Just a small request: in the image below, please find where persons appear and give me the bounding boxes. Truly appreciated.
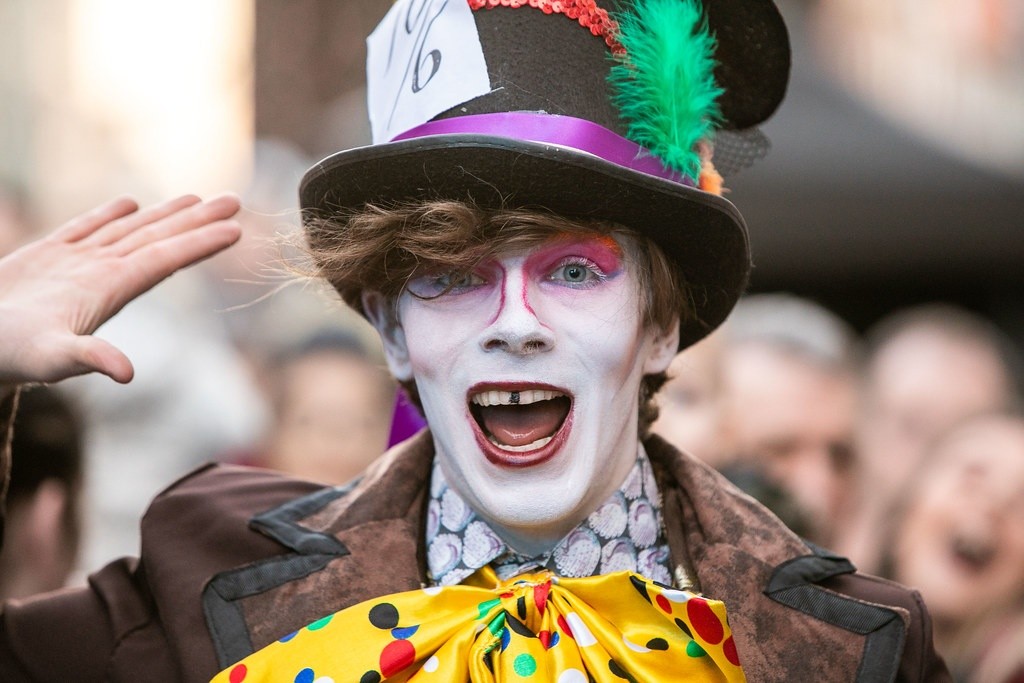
[0,0,955,683]
[0,288,1024,683]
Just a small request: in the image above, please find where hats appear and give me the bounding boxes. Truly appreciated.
[298,0,792,354]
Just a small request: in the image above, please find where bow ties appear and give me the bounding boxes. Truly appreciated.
[208,565,747,683]
